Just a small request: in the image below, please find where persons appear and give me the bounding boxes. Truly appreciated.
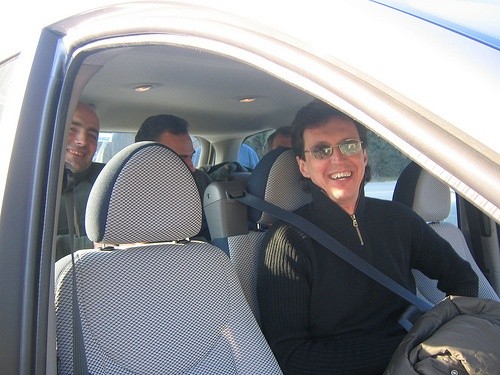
[54,100,107,262]
[264,126,295,155]
[135,114,214,244]
[255,98,480,375]
[192,142,259,173]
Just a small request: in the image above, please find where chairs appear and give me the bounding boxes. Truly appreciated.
[391,160,500,306]
[55,140,285,375]
[204,146,317,330]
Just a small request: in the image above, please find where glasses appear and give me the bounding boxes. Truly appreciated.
[303,139,364,161]
